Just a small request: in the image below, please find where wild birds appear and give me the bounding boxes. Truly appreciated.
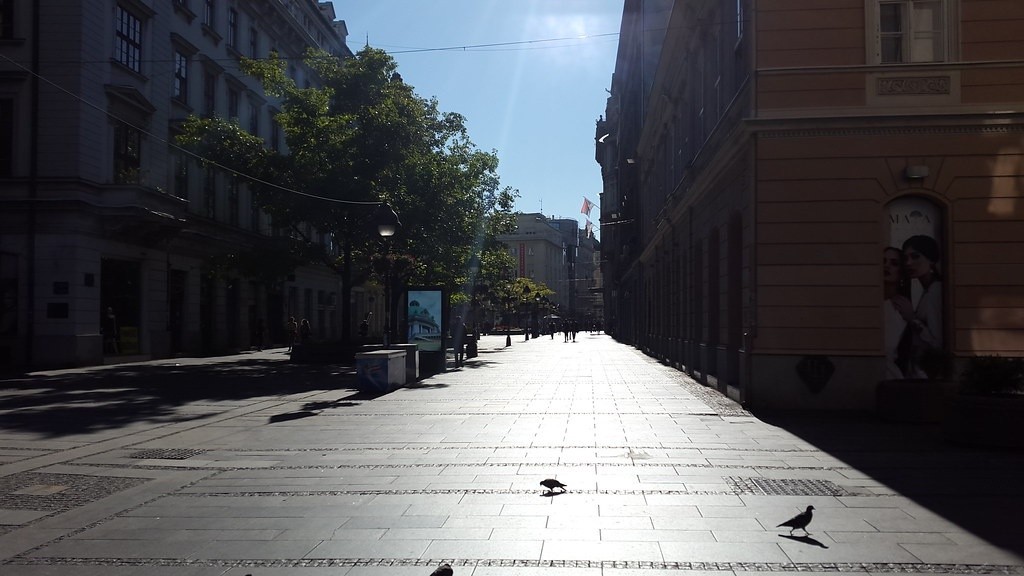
[430,563,453,576]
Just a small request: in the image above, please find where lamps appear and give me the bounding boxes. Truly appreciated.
[905,165,930,179]
[660,90,671,102]
[626,150,637,164]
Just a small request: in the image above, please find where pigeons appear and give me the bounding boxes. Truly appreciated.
[776,505,816,535]
[540,479,567,493]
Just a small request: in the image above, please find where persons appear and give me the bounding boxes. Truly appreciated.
[883,234,944,378]
[584,319,602,333]
[359,319,368,338]
[287,317,311,351]
[549,321,563,340]
[449,315,467,368]
[563,318,578,343]
[101,307,119,355]
[254,318,265,352]
